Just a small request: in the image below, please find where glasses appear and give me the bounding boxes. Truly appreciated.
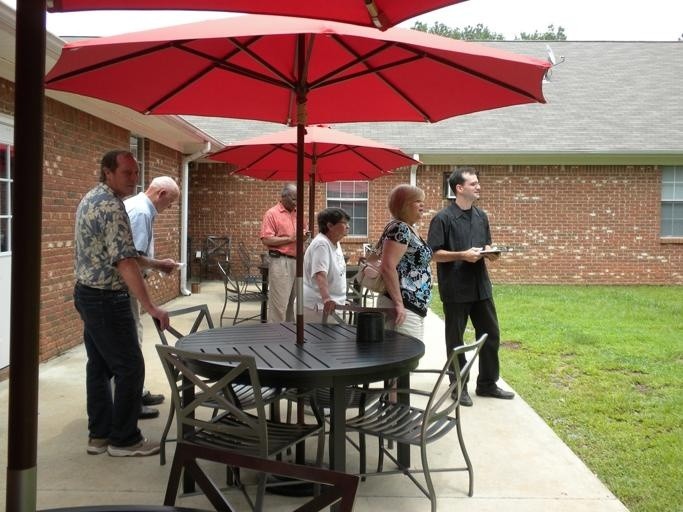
[338,221,347,226]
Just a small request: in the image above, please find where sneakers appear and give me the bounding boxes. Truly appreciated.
[107,436,160,457]
[87,436,107,455]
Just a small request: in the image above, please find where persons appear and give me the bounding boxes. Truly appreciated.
[259,183,311,324]
[124,176,181,418]
[71,150,170,458]
[427,166,516,407]
[375,183,435,408]
[303,206,352,418]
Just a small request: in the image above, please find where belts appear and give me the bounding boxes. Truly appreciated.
[280,253,296,259]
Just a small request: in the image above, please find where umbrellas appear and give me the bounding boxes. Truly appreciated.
[202,123,422,237]
[43,14,552,345]
[47,0,473,34]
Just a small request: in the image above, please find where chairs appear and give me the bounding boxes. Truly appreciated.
[152,304,289,486]
[347,332,487,512]
[217,261,269,327]
[286,300,395,463]
[155,344,326,512]
[162,441,361,512]
[235,241,263,294]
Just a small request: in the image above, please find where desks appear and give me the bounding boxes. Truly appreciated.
[174,322,425,474]
[258,263,363,325]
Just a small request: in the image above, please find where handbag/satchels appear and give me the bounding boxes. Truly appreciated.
[355,220,401,294]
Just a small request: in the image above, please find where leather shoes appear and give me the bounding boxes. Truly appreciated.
[139,407,159,418]
[476,384,514,399]
[141,392,164,405]
[451,385,473,407]
